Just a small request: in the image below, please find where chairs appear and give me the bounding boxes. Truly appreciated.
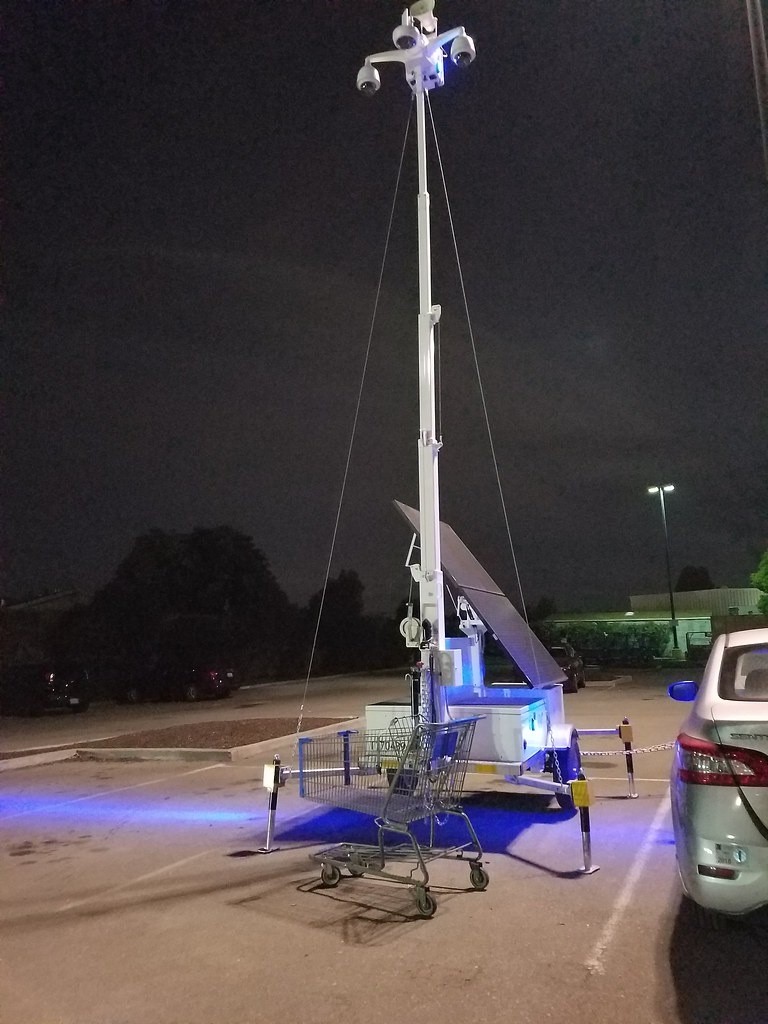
[745,669,768,699]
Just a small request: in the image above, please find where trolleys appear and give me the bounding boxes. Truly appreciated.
[297,712,490,919]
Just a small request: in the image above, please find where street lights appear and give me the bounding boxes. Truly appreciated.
[646,479,685,658]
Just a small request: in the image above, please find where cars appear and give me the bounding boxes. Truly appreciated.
[665,626,767,919]
[0,664,90,720]
[513,642,585,693]
[116,665,233,701]
[576,648,610,668]
